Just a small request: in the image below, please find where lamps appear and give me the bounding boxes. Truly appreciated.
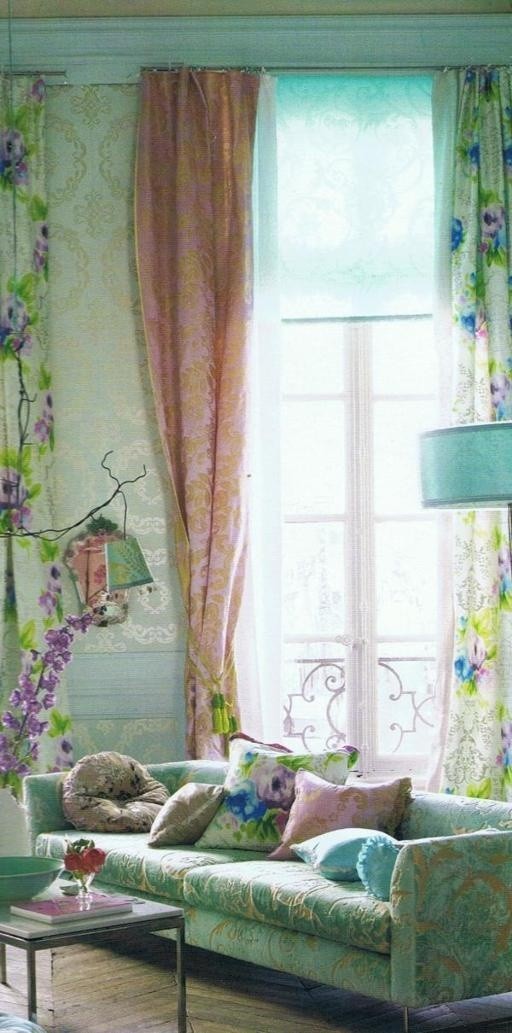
[410,421,512,558]
[103,489,154,594]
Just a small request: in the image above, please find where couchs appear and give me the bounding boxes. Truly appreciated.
[21,756,512,1033]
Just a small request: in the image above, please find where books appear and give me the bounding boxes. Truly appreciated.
[9,890,134,926]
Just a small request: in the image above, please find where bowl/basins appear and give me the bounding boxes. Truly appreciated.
[0,856,68,902]
